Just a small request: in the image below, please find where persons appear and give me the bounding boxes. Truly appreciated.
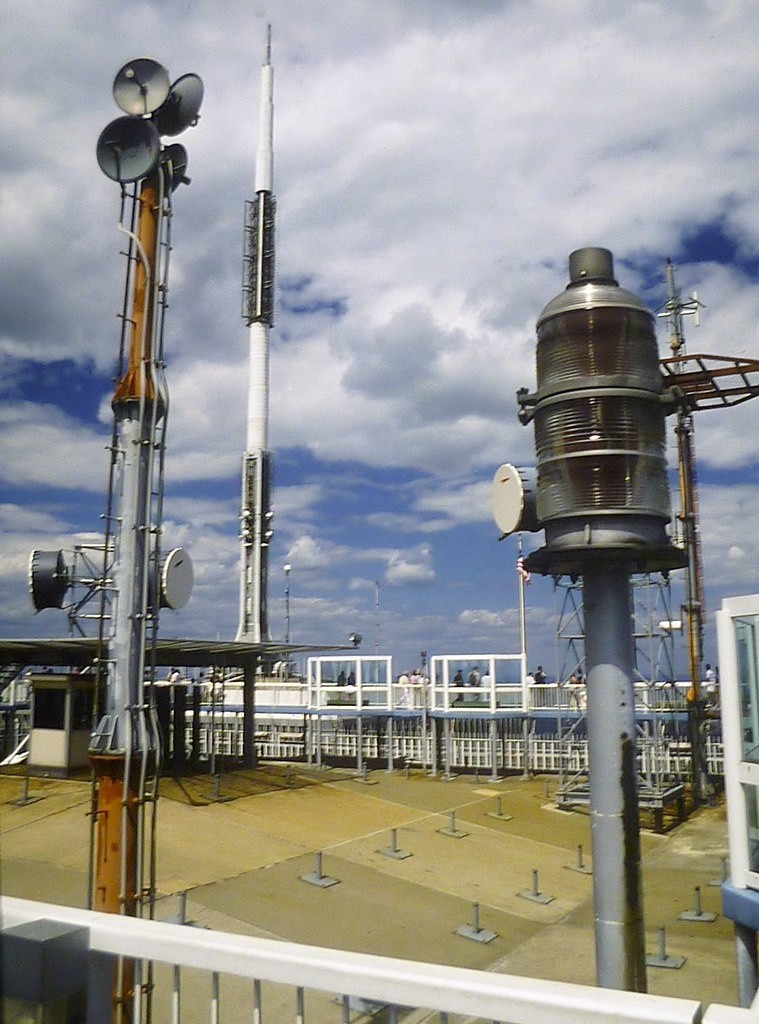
[702,664,717,704]
[26,659,587,707]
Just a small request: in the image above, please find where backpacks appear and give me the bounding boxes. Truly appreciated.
[535,671,543,683]
[468,672,475,686]
